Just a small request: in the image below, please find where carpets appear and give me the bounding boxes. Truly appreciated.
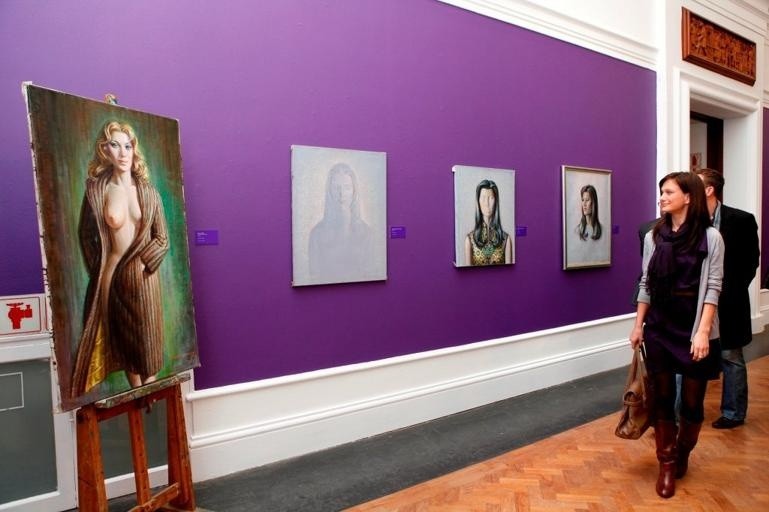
[61,321,769,512]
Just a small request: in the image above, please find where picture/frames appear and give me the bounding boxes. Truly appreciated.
[561,163,613,270]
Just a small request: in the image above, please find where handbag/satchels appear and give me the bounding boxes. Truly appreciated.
[615,342,651,439]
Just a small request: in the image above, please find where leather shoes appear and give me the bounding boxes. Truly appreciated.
[712,416,744,428]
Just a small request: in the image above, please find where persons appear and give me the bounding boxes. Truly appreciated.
[464,179,513,265]
[572,185,607,260]
[632,202,683,438]
[694,168,760,430]
[69,119,170,399]
[629,171,725,498]
[309,160,373,282]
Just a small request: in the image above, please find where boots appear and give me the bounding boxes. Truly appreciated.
[654,417,703,498]
